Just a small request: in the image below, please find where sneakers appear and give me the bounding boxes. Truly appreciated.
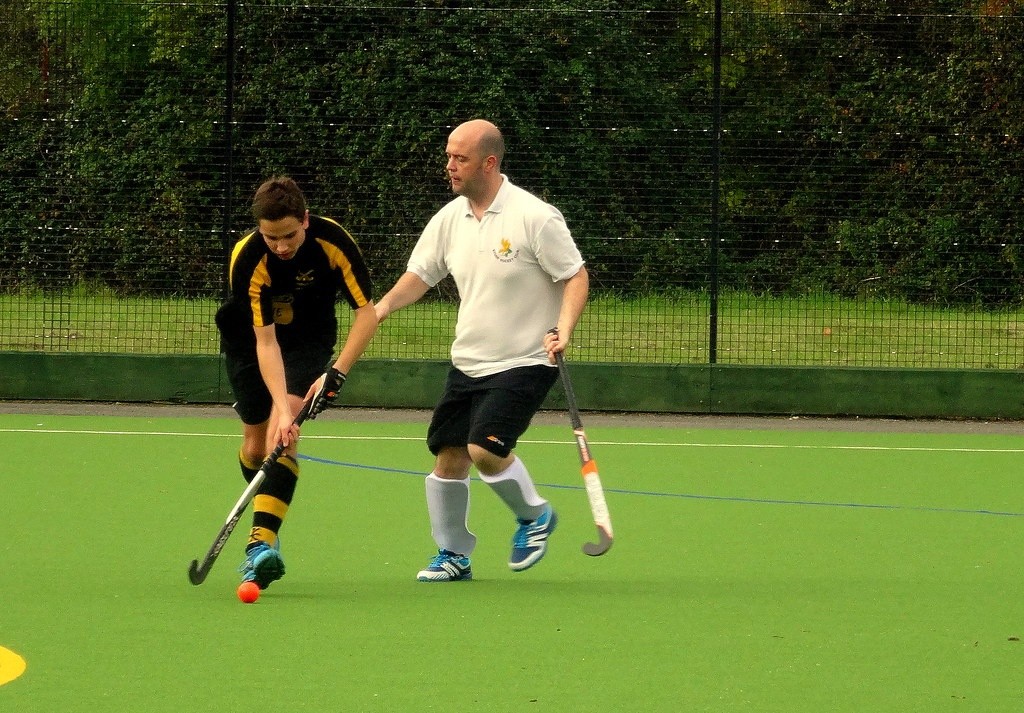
[238,541,286,589]
[506,502,557,571]
[416,549,472,581]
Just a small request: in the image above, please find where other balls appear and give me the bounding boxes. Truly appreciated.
[237,582,262,602]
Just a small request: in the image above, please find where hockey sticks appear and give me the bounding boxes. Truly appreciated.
[549,327,614,556]
[188,399,312,587]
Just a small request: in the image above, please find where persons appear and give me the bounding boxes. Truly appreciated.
[213,177,378,590]
[373,119,589,579]
